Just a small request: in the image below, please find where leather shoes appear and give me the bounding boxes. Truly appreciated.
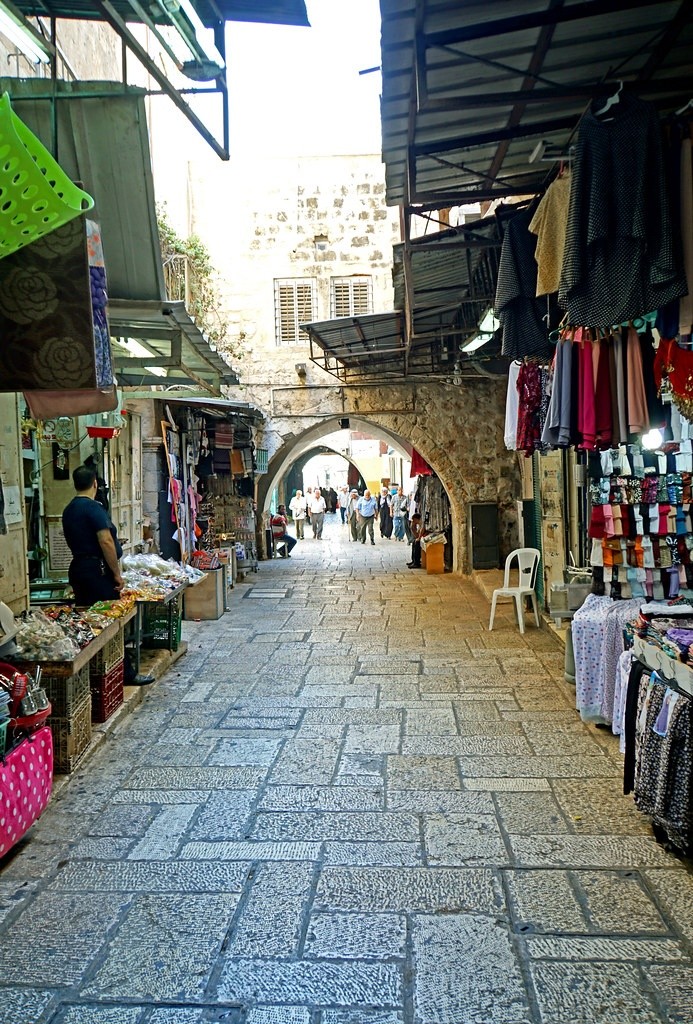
[125,673,155,686]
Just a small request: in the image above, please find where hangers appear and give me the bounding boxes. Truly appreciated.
[524,355,552,370]
[675,98,693,115]
[558,319,633,342]
[593,78,624,122]
[563,146,576,174]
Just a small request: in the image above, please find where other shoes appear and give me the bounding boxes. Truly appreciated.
[297,536,299,539]
[399,538,404,542]
[282,550,291,557]
[381,534,383,538]
[406,561,414,565]
[371,541,375,545]
[277,549,284,556]
[408,563,420,568]
[361,540,365,544]
[317,536,322,540]
[313,534,316,538]
[300,536,304,539]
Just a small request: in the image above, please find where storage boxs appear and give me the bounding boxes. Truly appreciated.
[38,662,90,717]
[88,625,124,677]
[143,592,182,652]
[46,695,93,775]
[184,568,224,620]
[91,661,123,723]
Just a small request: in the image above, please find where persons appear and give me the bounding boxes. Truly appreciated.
[271,504,297,557]
[289,486,338,540]
[62,465,125,607]
[338,484,432,569]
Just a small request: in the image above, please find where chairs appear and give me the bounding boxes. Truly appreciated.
[489,548,540,634]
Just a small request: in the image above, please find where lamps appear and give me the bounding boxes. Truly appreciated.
[338,417,350,429]
[295,363,307,377]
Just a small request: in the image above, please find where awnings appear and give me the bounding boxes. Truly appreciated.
[299,309,442,368]
[106,297,241,387]
[1,76,167,300]
[379,1,693,206]
[392,206,530,308]
[1,0,310,28]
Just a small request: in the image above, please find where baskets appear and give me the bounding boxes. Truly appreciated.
[0,89,95,258]
